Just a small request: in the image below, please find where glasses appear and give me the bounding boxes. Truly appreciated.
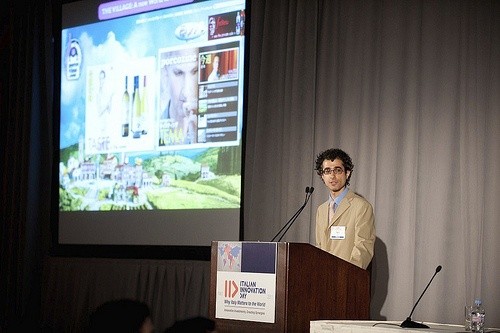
[322,167,347,175]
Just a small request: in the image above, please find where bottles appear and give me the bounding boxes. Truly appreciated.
[471,299,486,333]
[121,74,150,139]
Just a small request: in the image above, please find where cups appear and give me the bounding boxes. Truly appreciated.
[465,305,473,331]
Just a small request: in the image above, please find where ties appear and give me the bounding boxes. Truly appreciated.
[329,201,335,225]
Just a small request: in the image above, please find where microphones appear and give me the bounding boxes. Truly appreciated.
[400,265,442,328]
[270,187,314,242]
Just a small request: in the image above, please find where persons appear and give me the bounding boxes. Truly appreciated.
[209,16,217,36]
[82,297,155,333]
[207,54,222,82]
[162,315,216,333]
[159,47,199,142]
[315,148,375,270]
